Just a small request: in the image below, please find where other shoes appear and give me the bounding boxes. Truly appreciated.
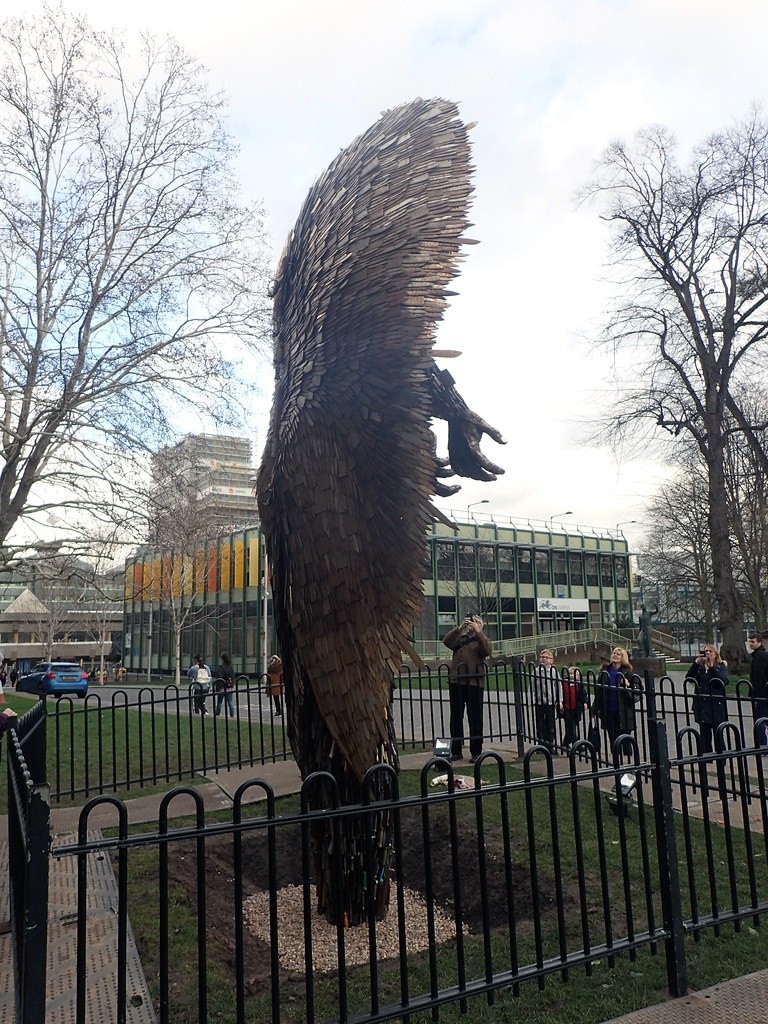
[449,753,463,761]
[274,712,282,717]
[469,755,478,763]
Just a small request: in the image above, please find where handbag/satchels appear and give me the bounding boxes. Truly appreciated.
[588,722,601,752]
[622,672,640,704]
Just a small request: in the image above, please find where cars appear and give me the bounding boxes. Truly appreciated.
[15,663,88,699]
[103,665,126,675]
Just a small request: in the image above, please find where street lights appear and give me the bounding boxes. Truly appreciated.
[468,500,489,519]
[551,512,573,528]
[617,521,636,535]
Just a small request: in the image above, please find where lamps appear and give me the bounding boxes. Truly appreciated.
[431,736,455,770]
[604,773,636,819]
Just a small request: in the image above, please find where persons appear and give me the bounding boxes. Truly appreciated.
[0,670,8,687]
[10,668,18,687]
[87,665,127,683]
[588,646,645,757]
[265,655,284,717]
[685,644,730,765]
[442,613,493,764]
[556,666,590,752]
[747,633,768,756]
[187,653,212,715]
[526,649,563,756]
[215,653,235,717]
[637,602,659,657]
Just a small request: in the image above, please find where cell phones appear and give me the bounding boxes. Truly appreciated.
[467,613,474,623]
[700,651,706,657]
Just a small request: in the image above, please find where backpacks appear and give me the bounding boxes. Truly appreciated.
[195,665,211,684]
[219,665,235,688]
